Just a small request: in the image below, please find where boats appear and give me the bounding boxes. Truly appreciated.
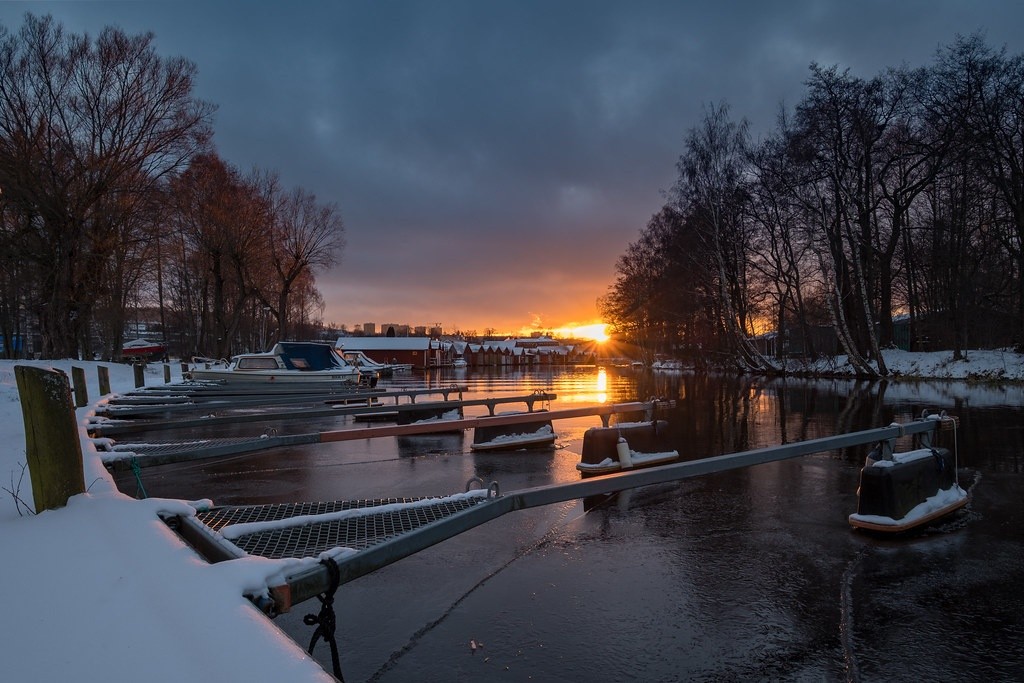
[186,341,413,384]
[652,361,695,369]
[575,420,680,476]
[110,339,166,361]
[470,408,559,453]
[610,360,644,368]
[324,398,384,409]
[847,448,984,532]
[452,359,467,367]
